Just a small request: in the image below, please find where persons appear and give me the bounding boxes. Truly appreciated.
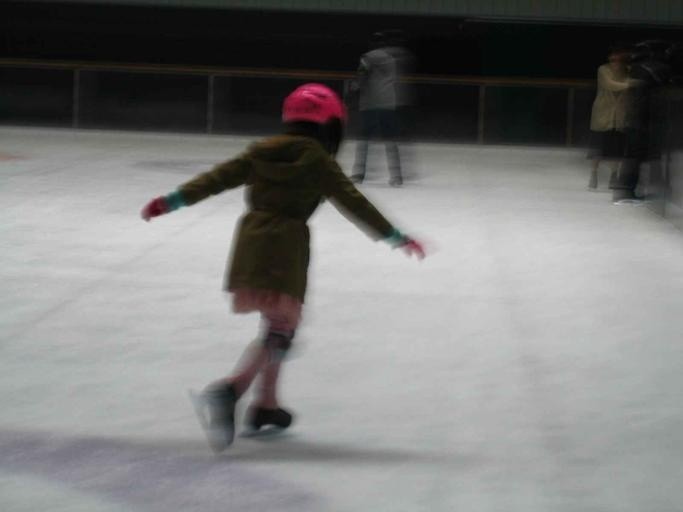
[611,38,665,203]
[585,43,644,190]
[139,81,428,450]
[345,31,403,187]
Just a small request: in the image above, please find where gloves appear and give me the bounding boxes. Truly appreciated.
[141,190,186,222]
[383,227,425,262]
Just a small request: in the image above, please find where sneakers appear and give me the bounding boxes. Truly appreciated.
[243,405,292,427]
[389,178,403,186]
[204,379,235,443]
[348,174,364,181]
[588,175,644,201]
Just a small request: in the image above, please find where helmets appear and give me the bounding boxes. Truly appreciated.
[281,83,351,131]
